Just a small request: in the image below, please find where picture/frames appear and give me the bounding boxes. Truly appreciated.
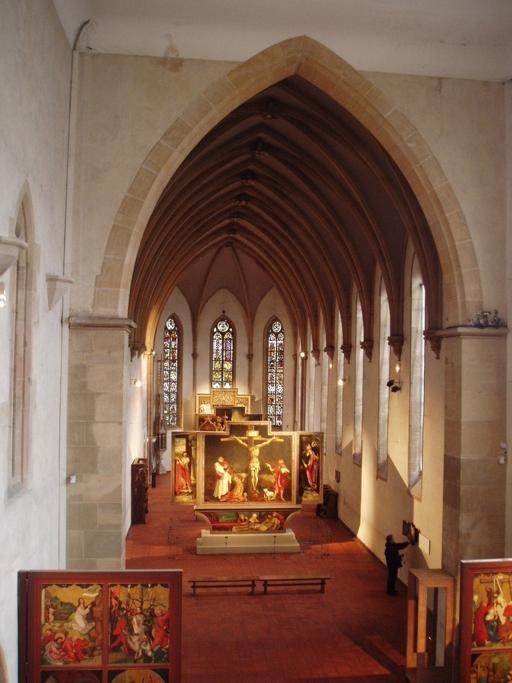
[195,422,301,512]
[17,571,182,683]
[297,431,323,505]
[460,557,512,683]
[168,429,201,507]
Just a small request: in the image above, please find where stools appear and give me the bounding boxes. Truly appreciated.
[406,566,455,683]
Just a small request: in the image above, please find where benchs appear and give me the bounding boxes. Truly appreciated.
[188,576,256,596]
[257,575,330,594]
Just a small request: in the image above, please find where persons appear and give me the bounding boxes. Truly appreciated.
[45,589,169,664]
[231,434,280,490]
[265,458,290,502]
[213,456,232,499]
[472,592,512,647]
[175,451,192,493]
[208,420,225,431]
[384,534,411,596]
[299,441,312,491]
[219,460,248,502]
[302,446,319,490]
